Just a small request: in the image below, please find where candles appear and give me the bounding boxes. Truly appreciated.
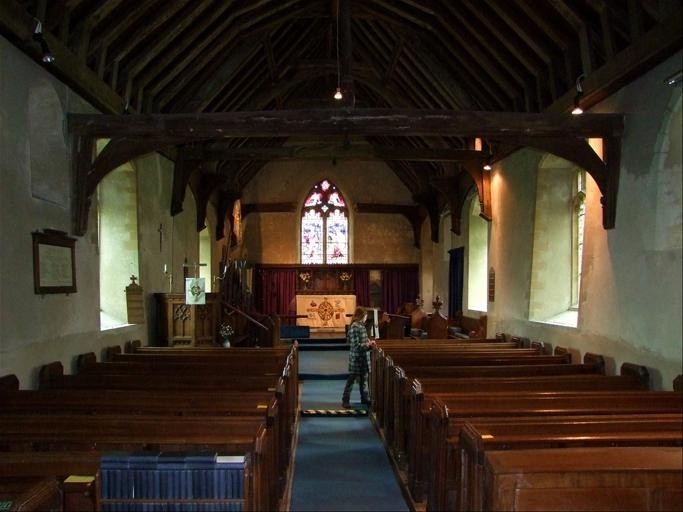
[223,265,228,275]
[164,264,168,274]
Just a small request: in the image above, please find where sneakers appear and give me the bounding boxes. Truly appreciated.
[340,402,350,408]
[361,400,369,404]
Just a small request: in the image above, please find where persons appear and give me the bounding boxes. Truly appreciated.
[342,305,376,410]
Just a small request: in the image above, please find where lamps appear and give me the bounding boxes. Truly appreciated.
[332,0,343,100]
[571,73,584,115]
[483,154,493,172]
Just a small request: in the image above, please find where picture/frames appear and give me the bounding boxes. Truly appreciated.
[30,227,78,296]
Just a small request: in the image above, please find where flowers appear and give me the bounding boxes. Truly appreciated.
[218,320,235,337]
